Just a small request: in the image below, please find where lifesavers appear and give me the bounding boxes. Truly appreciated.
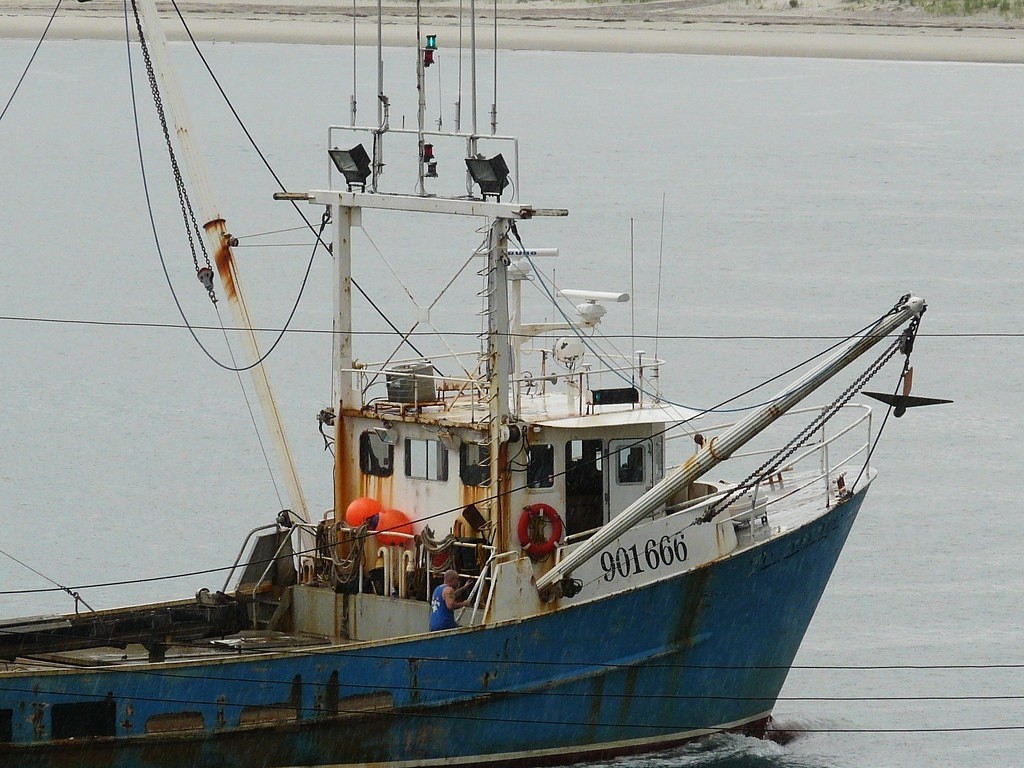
[518,503,562,555]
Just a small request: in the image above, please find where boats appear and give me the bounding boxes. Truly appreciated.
[0,1,955,767]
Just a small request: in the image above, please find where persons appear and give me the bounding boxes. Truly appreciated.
[429,569,473,633]
